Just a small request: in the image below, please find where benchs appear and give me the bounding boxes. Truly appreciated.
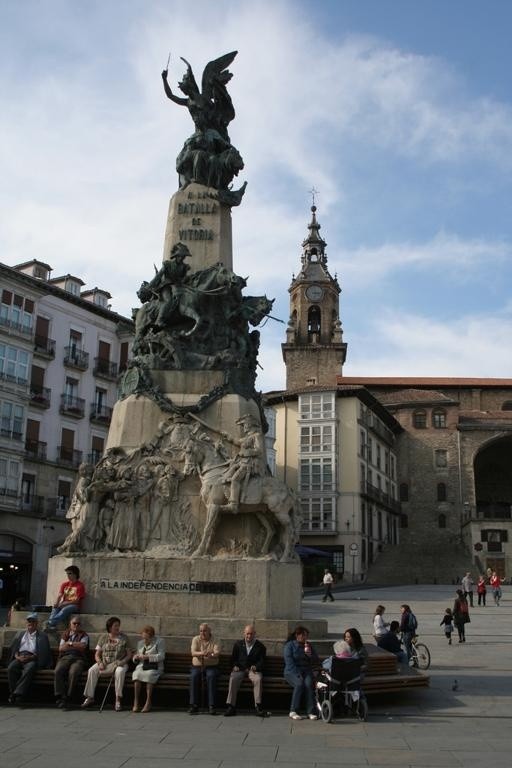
[0,655,430,692]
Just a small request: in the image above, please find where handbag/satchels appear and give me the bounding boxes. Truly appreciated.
[142,661,158,670]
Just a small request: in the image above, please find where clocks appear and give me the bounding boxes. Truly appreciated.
[306,285,323,301]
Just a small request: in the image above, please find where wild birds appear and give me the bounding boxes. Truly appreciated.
[452,678,458,691]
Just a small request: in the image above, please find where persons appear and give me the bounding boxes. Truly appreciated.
[400,604,418,659]
[131,626,166,713]
[441,567,506,645]
[81,617,131,711]
[373,605,387,643]
[54,617,89,704]
[319,569,334,602]
[224,624,264,716]
[161,70,214,134]
[283,624,368,720]
[220,415,263,514]
[57,447,180,553]
[144,241,192,328]
[188,623,220,715]
[7,614,51,703]
[44,565,85,632]
[378,621,408,665]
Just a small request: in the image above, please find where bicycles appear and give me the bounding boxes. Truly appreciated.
[398,630,431,670]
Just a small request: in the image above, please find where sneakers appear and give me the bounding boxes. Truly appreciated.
[115,700,120,711]
[187,706,198,713]
[290,712,301,719]
[208,707,215,714]
[255,710,266,715]
[134,702,140,711]
[83,696,93,707]
[142,701,151,712]
[225,709,235,716]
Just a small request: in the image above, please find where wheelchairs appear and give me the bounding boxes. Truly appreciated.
[313,656,370,723]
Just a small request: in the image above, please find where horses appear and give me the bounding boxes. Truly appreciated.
[184,433,304,562]
[131,262,276,370]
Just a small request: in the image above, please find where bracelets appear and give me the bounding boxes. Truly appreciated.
[69,641,72,646]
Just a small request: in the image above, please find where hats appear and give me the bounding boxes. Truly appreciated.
[27,613,36,619]
[100,663,116,676]
